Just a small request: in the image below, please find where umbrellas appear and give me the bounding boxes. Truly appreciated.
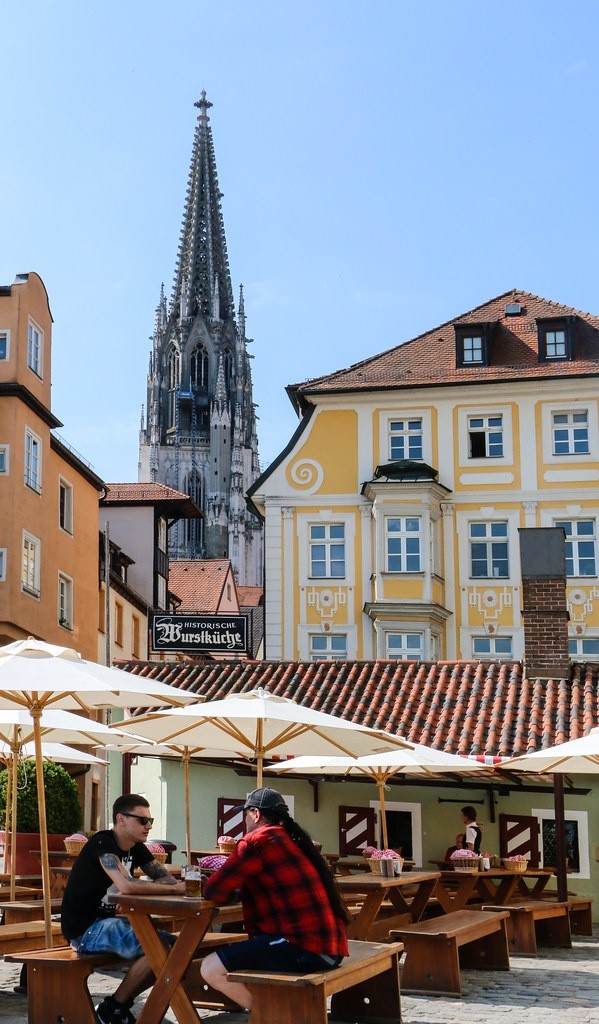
[0,635,599,952]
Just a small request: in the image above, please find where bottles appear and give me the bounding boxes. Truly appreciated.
[494,854,498,867]
[479,853,484,872]
[386,855,394,877]
[379,854,387,876]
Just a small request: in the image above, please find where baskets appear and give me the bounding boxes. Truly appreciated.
[131,853,168,871]
[448,856,482,873]
[199,868,218,896]
[63,839,88,854]
[502,858,529,873]
[218,842,237,853]
[366,857,404,875]
[315,844,323,853]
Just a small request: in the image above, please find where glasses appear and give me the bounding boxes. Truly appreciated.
[113,811,154,826]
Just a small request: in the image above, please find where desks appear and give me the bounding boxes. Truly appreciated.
[433,868,554,912]
[26,850,77,867]
[182,849,224,868]
[106,891,244,1024]
[50,866,73,899]
[331,871,442,941]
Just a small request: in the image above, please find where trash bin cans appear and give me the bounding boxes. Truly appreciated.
[147,840,177,864]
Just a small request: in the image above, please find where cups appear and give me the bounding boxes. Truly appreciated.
[180,864,195,882]
[184,866,202,898]
[393,861,401,877]
[483,858,489,870]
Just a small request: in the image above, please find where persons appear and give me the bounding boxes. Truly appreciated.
[388,843,413,872]
[445,834,465,861]
[60,794,208,1024]
[460,806,482,856]
[200,787,351,1014]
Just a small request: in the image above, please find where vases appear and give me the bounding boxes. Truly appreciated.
[63,840,87,854]
[365,858,404,876]
[153,852,168,866]
[363,852,372,858]
[218,841,236,852]
[503,861,529,872]
[449,857,481,874]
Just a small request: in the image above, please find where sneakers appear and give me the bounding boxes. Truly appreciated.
[93,1000,132,1024]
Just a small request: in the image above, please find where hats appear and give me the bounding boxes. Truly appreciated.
[232,786,288,813]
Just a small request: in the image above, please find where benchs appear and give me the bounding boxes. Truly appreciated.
[0,860,594,1024]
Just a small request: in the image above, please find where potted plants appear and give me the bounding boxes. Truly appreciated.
[0,759,83,875]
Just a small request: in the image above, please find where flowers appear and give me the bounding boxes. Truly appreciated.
[197,855,228,869]
[65,834,88,841]
[372,850,401,860]
[217,836,235,843]
[506,854,527,862]
[451,849,477,857]
[144,843,165,853]
[362,846,378,853]
